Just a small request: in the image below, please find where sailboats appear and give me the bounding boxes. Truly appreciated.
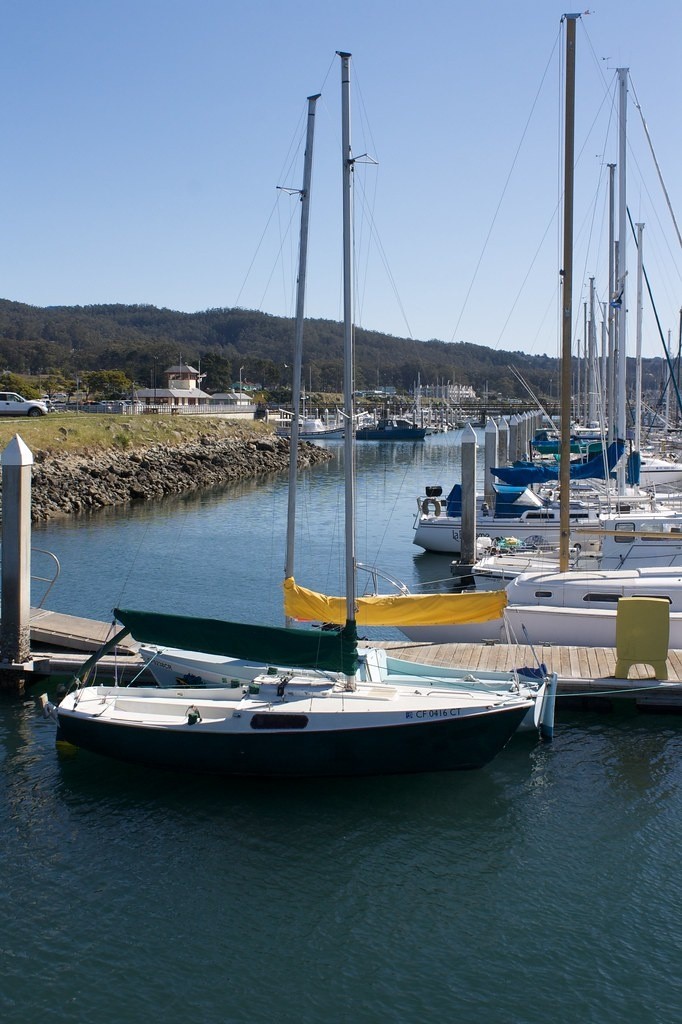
[53,46,535,779]
[390,9,682,650]
[108,90,562,744]
[272,370,503,441]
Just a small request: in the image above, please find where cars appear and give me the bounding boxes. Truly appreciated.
[0,392,48,417]
[37,398,143,412]
[53,392,67,398]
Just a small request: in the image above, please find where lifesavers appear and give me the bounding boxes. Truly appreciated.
[421,497,442,518]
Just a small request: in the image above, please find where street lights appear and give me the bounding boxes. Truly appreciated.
[153,355,159,397]
[240,365,245,405]
